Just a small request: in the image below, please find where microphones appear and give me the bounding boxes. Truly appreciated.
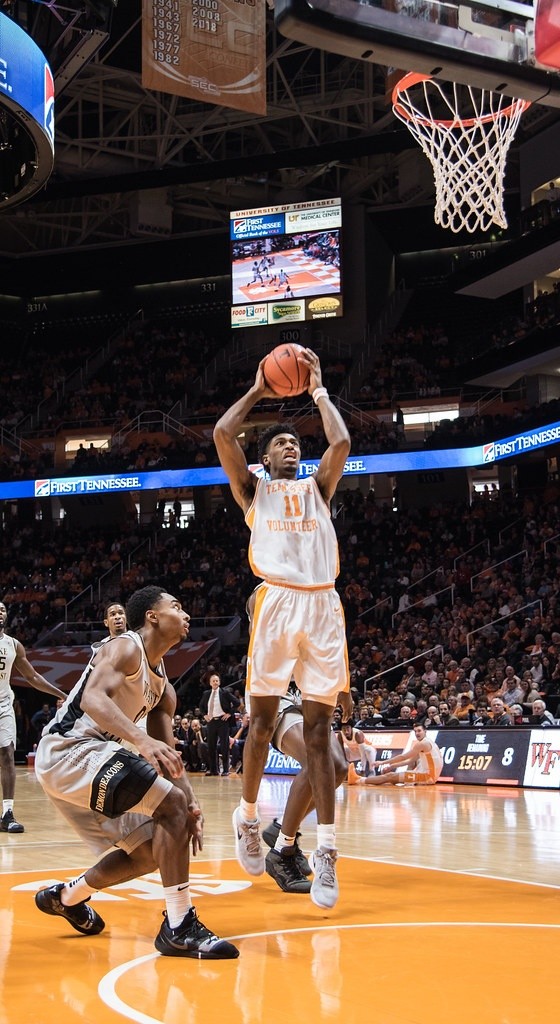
[474,717,482,726]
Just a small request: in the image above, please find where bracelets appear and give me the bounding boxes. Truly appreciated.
[312,387,329,404]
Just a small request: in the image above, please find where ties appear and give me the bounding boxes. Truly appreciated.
[208,689,216,720]
[195,733,200,745]
[184,731,188,741]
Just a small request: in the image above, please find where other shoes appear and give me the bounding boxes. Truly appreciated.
[221,772,230,776]
[235,761,244,774]
[205,772,219,776]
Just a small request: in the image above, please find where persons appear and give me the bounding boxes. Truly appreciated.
[0,602,68,834]
[0,284,560,647]
[104,603,168,758]
[172,654,250,776]
[245,582,354,893]
[214,348,351,908]
[348,724,443,785]
[199,675,241,776]
[13,699,63,749]
[234,234,339,298]
[547,182,560,216]
[337,720,376,777]
[35,588,240,959]
[335,467,560,726]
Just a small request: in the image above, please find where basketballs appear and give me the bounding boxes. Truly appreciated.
[263,342,310,397]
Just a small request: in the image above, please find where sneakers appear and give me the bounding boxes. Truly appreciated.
[0,808,25,833]
[264,844,313,894]
[262,816,312,876]
[308,844,338,911]
[347,761,361,786]
[152,904,240,960]
[35,883,106,935]
[231,804,266,876]
